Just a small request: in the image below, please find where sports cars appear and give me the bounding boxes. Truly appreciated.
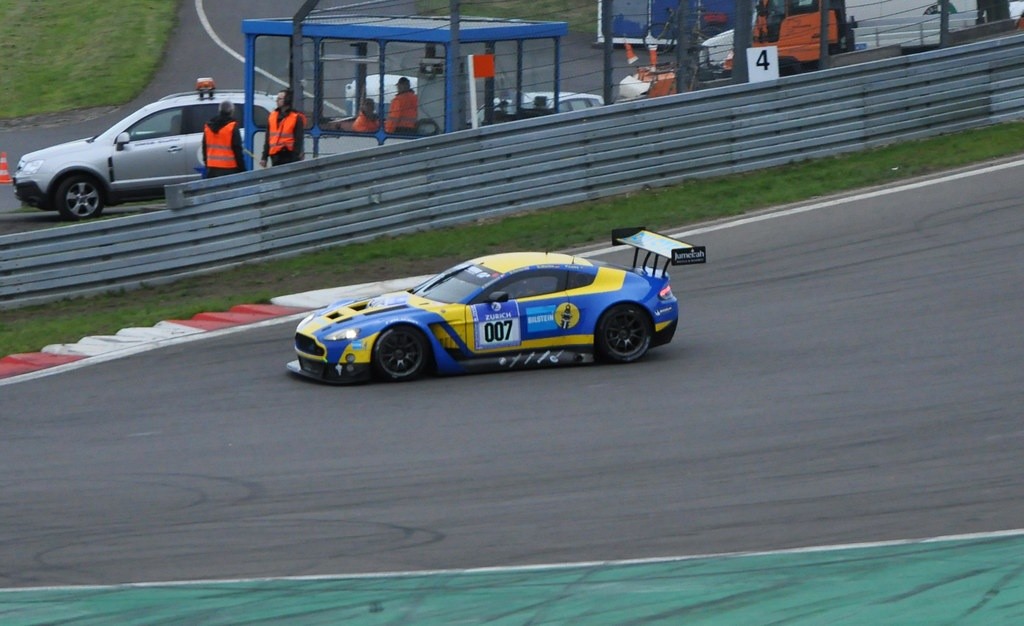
[284,224,707,387]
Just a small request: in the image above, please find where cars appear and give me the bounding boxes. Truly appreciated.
[466,89,606,126]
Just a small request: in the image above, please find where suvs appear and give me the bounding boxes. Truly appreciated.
[11,74,290,223]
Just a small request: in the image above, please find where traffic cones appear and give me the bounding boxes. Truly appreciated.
[0,151,13,183]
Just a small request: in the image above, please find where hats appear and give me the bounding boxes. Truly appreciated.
[395,77,410,86]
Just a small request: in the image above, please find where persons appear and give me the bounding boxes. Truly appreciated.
[384,77,419,135]
[201,101,245,179]
[259,90,307,169]
[351,99,380,133]
